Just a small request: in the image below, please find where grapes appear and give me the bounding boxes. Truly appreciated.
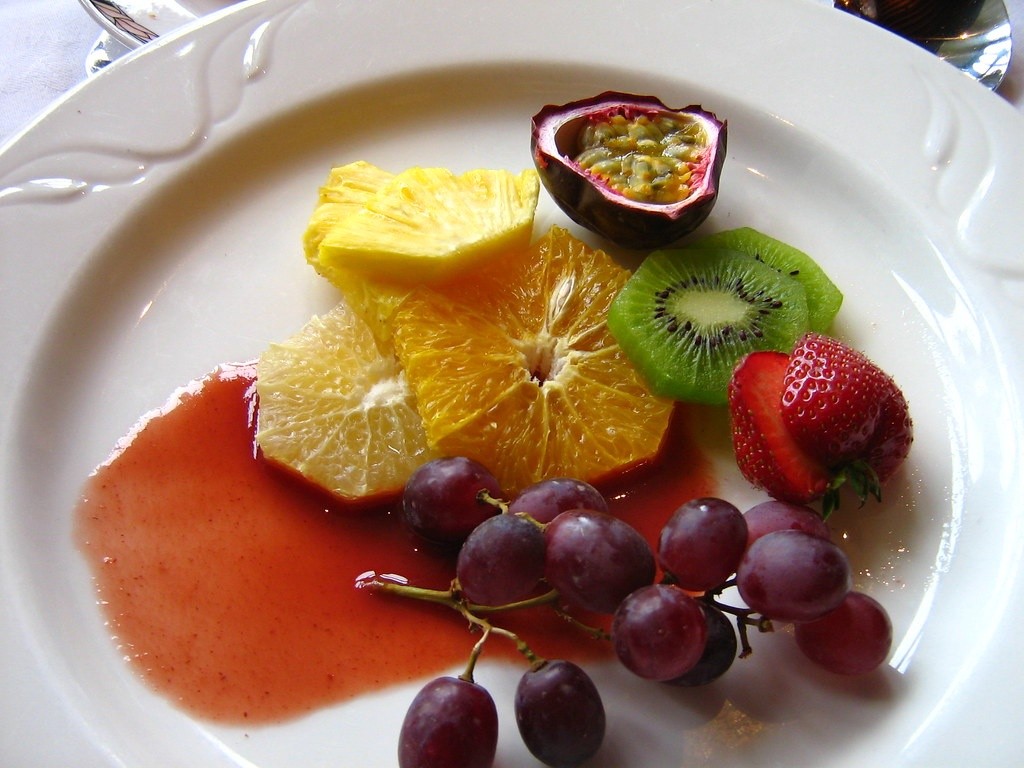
[398,453,892,768]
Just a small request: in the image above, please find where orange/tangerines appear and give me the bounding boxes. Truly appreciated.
[392,225,677,496]
[255,300,430,504]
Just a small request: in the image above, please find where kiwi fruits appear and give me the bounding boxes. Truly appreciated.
[607,245,812,405]
[680,227,844,333]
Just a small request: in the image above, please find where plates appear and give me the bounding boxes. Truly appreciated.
[0,0,1024,768]
[833,0,1014,94]
[76,0,248,52]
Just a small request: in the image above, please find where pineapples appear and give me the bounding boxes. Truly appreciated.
[300,159,540,336]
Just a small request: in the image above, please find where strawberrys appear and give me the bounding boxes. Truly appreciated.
[728,330,914,524]
